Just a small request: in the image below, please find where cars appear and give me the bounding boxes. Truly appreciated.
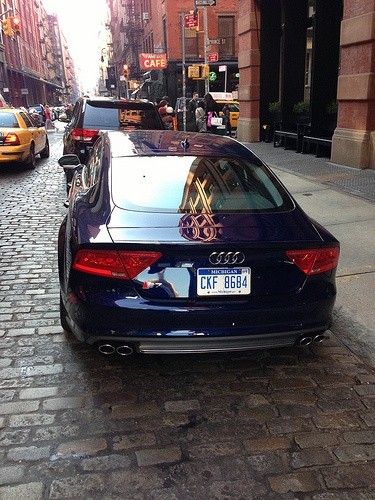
[216,100,240,128]
[55,129,343,358]
[0,106,50,171]
[27,104,68,128]
[58,95,166,194]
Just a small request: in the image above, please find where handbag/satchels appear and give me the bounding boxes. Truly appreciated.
[210,111,223,126]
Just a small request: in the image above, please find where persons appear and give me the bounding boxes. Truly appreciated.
[188,92,200,131]
[222,103,234,138]
[44,106,60,133]
[195,101,207,132]
[156,96,175,129]
[67,105,74,118]
[202,93,222,134]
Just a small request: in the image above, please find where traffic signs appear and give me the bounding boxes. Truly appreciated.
[193,0,217,7]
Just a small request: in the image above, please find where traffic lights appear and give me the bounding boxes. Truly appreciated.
[0,16,14,37]
[200,65,210,78]
[13,17,21,36]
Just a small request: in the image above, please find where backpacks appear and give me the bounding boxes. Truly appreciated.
[221,108,228,125]
